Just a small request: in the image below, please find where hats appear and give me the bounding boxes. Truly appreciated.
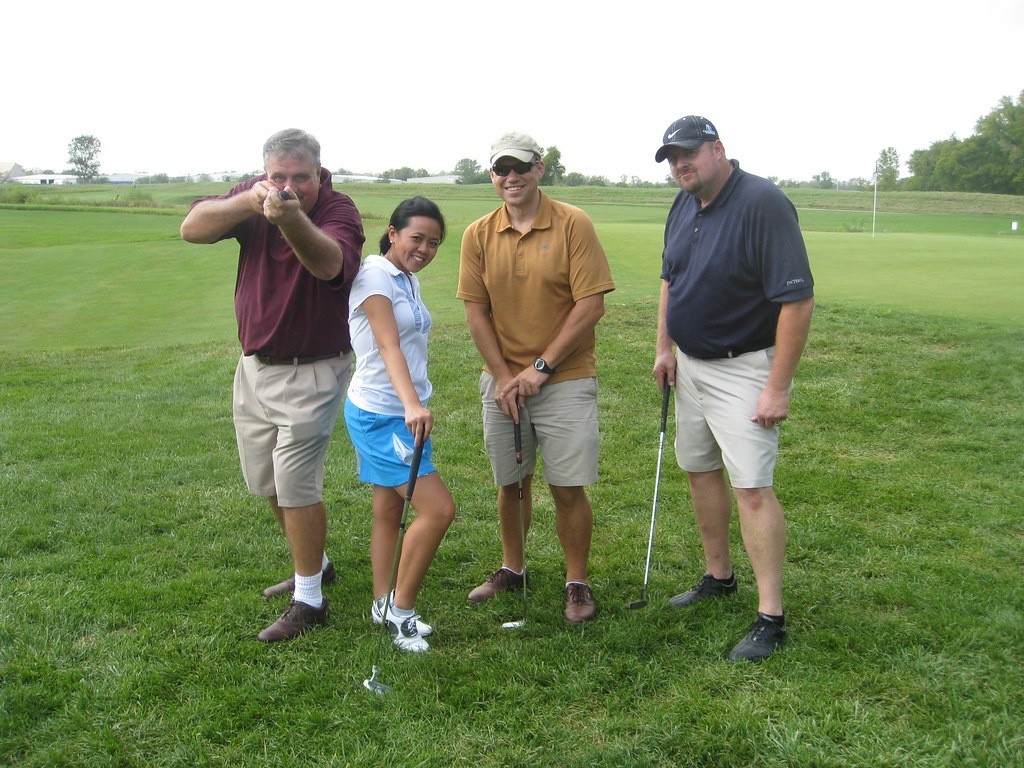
[655,115,719,163]
[488,131,542,168]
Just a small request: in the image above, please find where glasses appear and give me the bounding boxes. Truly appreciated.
[492,161,538,177]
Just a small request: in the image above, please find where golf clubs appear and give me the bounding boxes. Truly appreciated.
[501,394,529,630]
[363,428,425,696]
[622,374,671,612]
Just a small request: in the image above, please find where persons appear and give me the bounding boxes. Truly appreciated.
[457,127,617,625]
[342,192,456,655]
[653,115,815,674]
[180,125,370,647]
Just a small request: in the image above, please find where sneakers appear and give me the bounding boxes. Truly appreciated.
[256,596,330,643]
[669,573,737,609]
[263,561,336,598]
[562,583,596,625]
[728,612,789,664]
[467,570,530,602]
[371,601,432,637]
[372,594,429,653]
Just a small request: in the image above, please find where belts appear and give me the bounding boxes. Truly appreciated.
[255,344,353,366]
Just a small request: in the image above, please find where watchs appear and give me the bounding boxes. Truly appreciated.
[532,356,556,375]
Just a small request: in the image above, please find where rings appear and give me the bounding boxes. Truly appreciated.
[774,421,783,426]
[266,203,272,206]
